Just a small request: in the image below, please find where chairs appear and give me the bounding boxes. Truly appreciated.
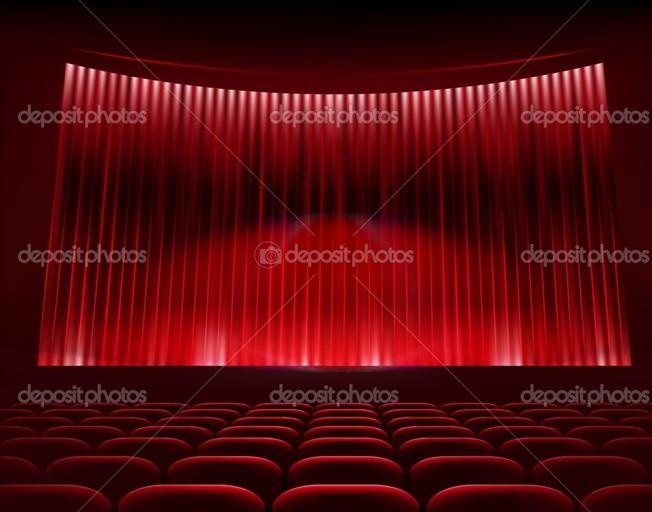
[0,399,652,511]
